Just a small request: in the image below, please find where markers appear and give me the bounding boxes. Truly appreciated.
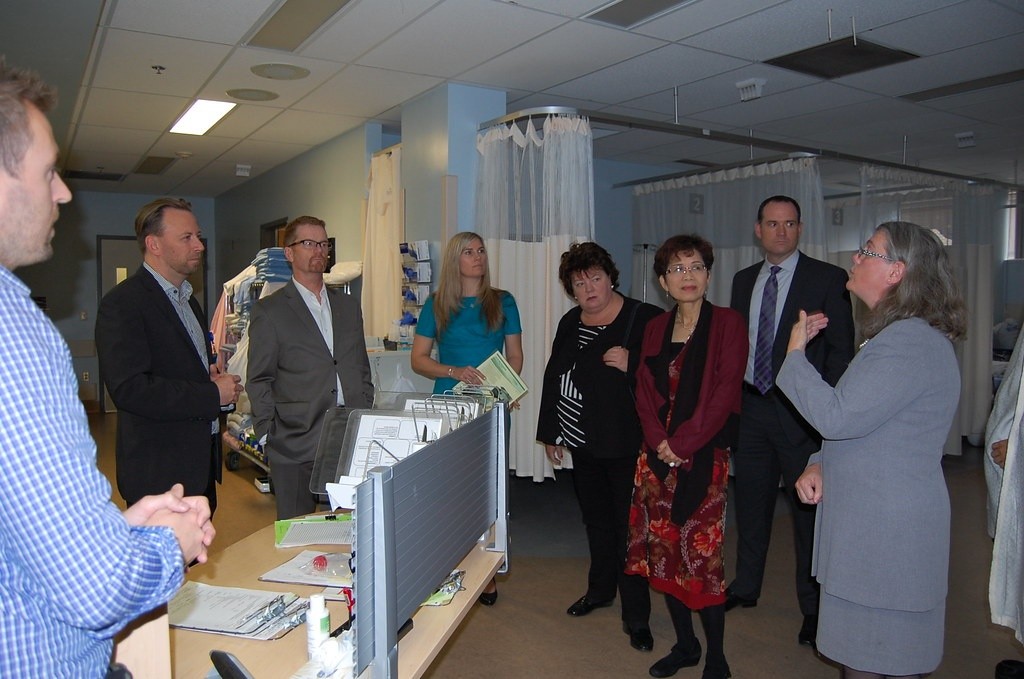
[305,516,336,521]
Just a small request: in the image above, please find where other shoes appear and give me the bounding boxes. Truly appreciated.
[649,638,703,678]
[702,653,731,679]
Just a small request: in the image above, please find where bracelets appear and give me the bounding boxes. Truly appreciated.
[448,368,452,377]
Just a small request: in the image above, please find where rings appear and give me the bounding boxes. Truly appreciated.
[670,462,674,466]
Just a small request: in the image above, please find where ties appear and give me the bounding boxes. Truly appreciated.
[753,266,782,395]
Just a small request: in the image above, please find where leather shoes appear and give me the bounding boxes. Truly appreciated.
[623,618,655,653]
[478,577,498,606]
[567,594,614,616]
[720,587,758,612]
[798,615,820,645]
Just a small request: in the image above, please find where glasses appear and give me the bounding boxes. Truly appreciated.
[663,262,708,276]
[858,243,895,262]
[287,239,330,249]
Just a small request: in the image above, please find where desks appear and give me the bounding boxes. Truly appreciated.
[111,500,506,679]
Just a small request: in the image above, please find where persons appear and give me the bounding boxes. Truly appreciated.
[95,196,245,567]
[0,61,216,679]
[536,241,665,652]
[246,214,375,521]
[624,233,750,679]
[412,232,523,414]
[724,195,854,644]
[984,323,1024,679]
[776,221,963,679]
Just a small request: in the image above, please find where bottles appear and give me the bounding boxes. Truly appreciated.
[306,594,332,659]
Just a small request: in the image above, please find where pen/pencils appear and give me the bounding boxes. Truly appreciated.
[343,586,354,612]
[210,331,216,354]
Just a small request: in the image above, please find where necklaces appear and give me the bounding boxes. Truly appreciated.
[677,305,694,330]
[469,302,474,308]
